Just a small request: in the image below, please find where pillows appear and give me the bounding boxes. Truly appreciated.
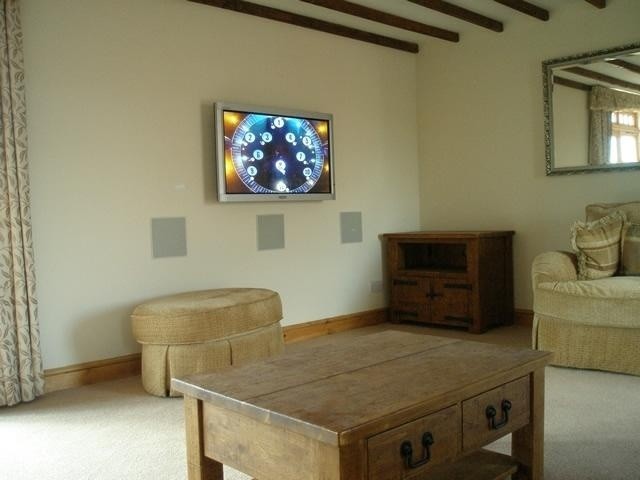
[619,222,640,276]
[568,213,626,280]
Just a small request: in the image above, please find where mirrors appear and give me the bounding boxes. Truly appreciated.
[542,42,640,175]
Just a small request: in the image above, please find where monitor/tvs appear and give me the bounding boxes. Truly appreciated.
[214,101,337,204]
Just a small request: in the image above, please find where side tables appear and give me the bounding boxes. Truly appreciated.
[131,288,286,397]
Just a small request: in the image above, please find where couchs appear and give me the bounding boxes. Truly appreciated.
[531,200,640,376]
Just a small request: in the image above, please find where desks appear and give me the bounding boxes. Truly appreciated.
[172,328,555,480]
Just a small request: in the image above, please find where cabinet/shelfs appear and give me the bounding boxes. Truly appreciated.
[377,230,516,335]
